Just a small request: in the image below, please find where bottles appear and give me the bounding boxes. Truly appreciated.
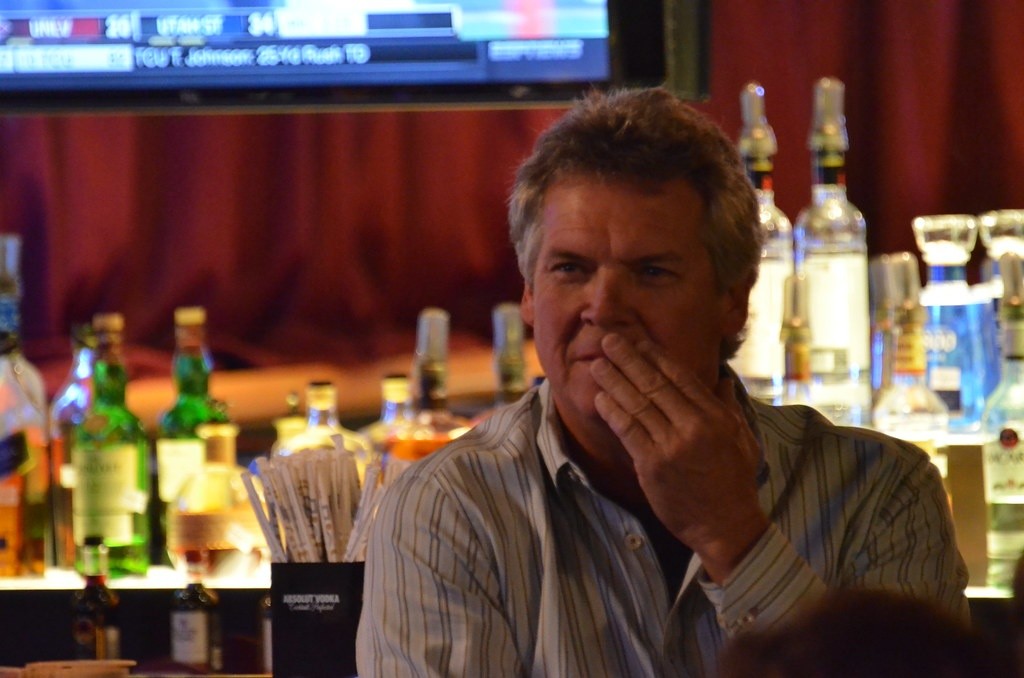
[162,549,226,671]
[979,252,1024,588]
[51,323,99,570]
[269,301,525,490]
[155,305,230,563]
[723,76,873,430]
[0,293,51,577]
[71,534,123,661]
[870,210,1024,431]
[71,313,152,579]
[167,422,270,579]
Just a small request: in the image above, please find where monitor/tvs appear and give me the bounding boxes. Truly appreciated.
[0,0,711,116]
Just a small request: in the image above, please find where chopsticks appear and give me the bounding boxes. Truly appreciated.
[238,434,412,564]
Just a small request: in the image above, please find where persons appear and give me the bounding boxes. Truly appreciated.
[352,87,1024,678]
